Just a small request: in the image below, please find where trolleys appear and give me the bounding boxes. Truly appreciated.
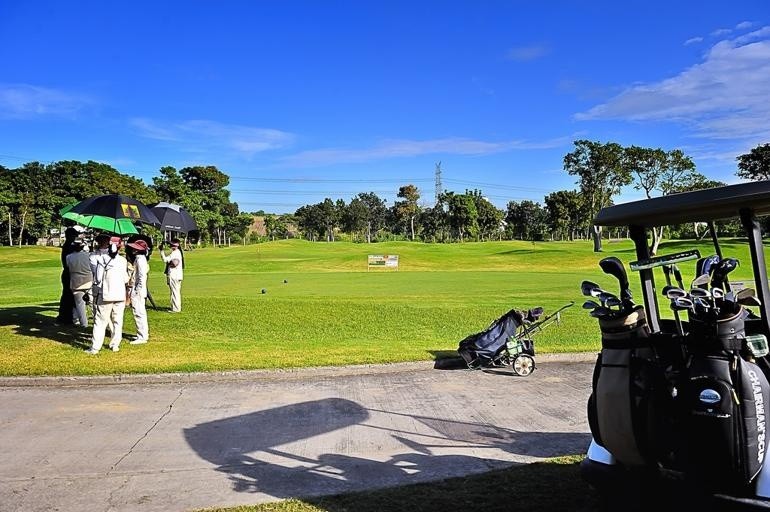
[459,301,573,376]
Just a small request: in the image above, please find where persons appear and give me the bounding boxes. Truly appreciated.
[159,241,184,316]
[55,225,153,356]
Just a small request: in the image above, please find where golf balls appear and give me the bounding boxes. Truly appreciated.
[262,289,266,293]
[284,279,288,283]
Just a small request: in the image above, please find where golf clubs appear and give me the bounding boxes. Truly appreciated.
[662,254,763,318]
[581,256,635,318]
[82,245,122,302]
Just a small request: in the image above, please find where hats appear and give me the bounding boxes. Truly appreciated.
[109,236,121,247]
[166,239,181,247]
[64,228,81,236]
[71,239,85,246]
[128,239,148,251]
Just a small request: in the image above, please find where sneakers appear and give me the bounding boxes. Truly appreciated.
[130,338,148,345]
[84,347,99,354]
[112,345,119,352]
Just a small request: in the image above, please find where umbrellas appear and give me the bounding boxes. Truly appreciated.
[57,204,139,236]
[68,193,162,236]
[145,200,199,238]
